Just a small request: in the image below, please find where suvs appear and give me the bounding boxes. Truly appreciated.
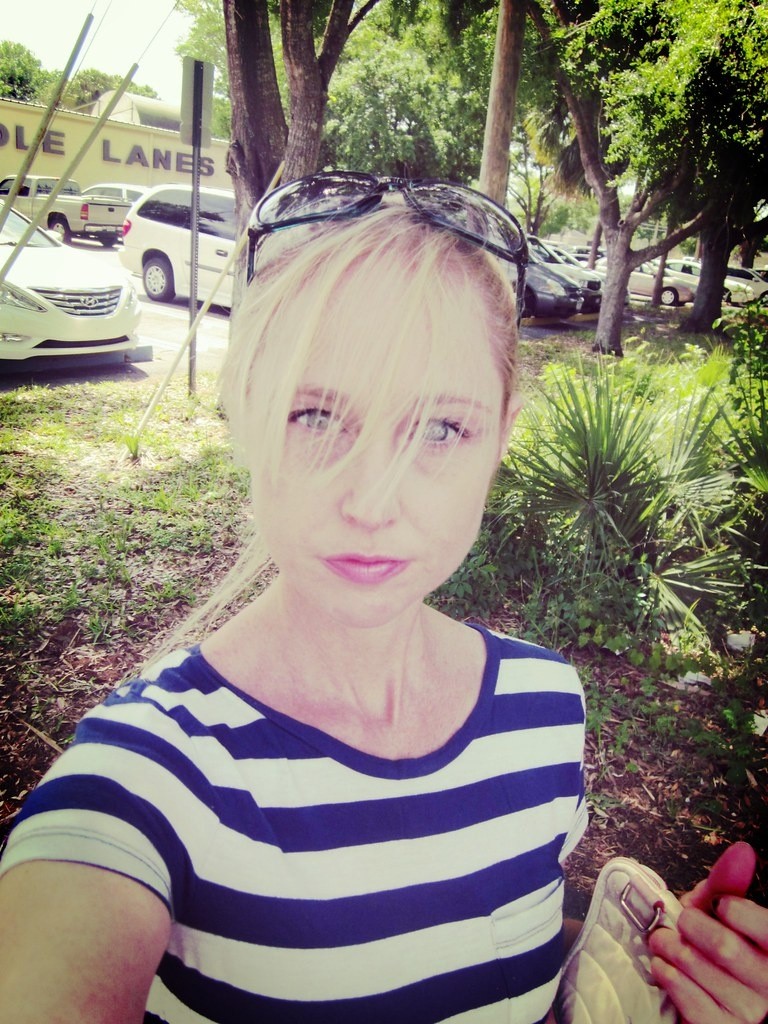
[119,184,235,310]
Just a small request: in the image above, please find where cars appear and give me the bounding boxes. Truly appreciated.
[0,199,143,363]
[81,183,148,199]
[520,235,767,315]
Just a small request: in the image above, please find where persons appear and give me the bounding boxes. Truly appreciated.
[0,168,768,1024]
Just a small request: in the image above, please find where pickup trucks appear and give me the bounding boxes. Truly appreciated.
[0,175,131,244]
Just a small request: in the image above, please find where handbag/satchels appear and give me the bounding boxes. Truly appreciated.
[557,857,682,1024]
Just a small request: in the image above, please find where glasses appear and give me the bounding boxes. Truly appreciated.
[246,170,538,324]
